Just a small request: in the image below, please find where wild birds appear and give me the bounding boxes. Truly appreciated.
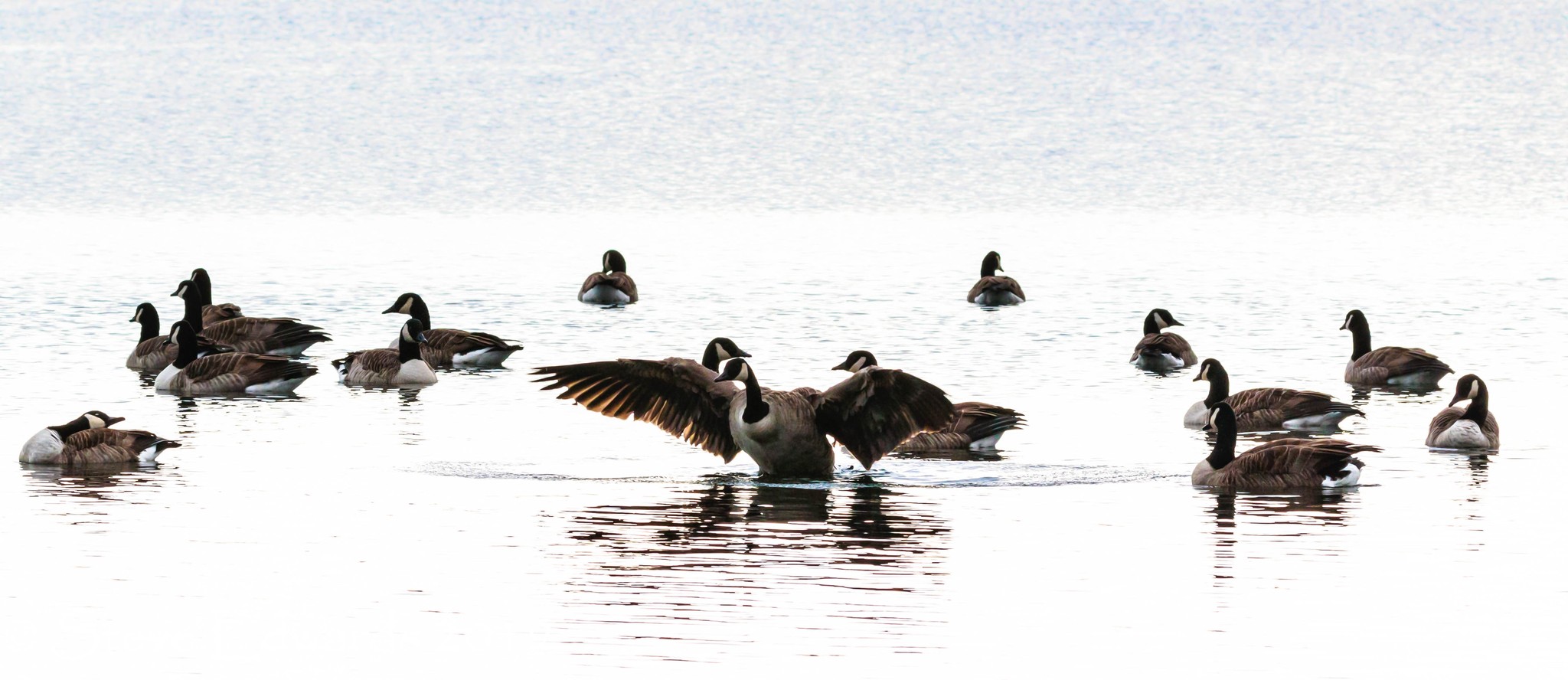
[171,280,236,358]
[1128,308,1198,370]
[967,251,1026,306]
[832,351,1028,449]
[381,292,524,366]
[1183,359,1369,430]
[529,355,957,483]
[125,301,239,369]
[191,267,334,356]
[578,250,639,304]
[1192,402,1386,489]
[1425,373,1500,450]
[1339,309,1456,385]
[331,318,438,384]
[701,338,751,372]
[155,321,321,394]
[19,410,183,465]
